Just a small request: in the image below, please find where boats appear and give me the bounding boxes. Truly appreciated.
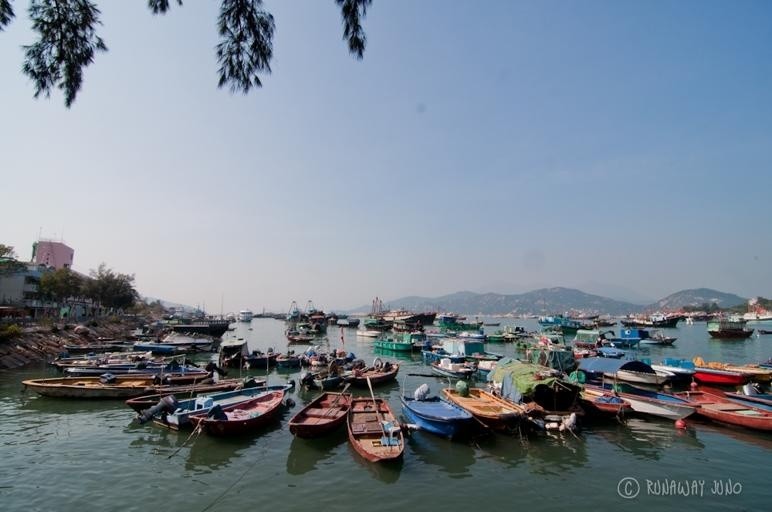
[422,333,504,370]
[621,311,685,326]
[154,381,292,427]
[594,315,617,326]
[438,312,466,324]
[152,330,213,352]
[285,301,310,330]
[336,313,349,318]
[470,316,484,327]
[539,314,595,329]
[326,312,338,326]
[63,360,207,377]
[302,349,354,366]
[364,297,392,331]
[442,383,526,433]
[239,310,252,323]
[688,310,715,321]
[563,357,702,422]
[63,344,132,351]
[289,391,352,438]
[143,314,228,338]
[125,379,244,417]
[52,351,208,365]
[133,343,177,351]
[398,384,473,438]
[276,350,300,368]
[226,313,236,324]
[347,400,404,463]
[190,390,285,438]
[288,323,316,342]
[248,347,278,367]
[219,340,248,371]
[621,328,676,345]
[575,329,641,347]
[644,363,771,433]
[435,316,483,329]
[757,329,772,335]
[558,378,631,419]
[300,359,368,388]
[485,329,518,342]
[573,350,596,358]
[344,357,399,388]
[651,364,697,381]
[597,347,624,358]
[572,310,600,319]
[374,337,415,351]
[300,301,325,334]
[23,372,212,397]
[394,319,424,333]
[486,357,584,443]
[485,323,499,326]
[432,359,472,382]
[683,357,772,385]
[708,318,754,338]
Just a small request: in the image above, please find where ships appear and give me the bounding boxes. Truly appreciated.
[367,296,438,325]
